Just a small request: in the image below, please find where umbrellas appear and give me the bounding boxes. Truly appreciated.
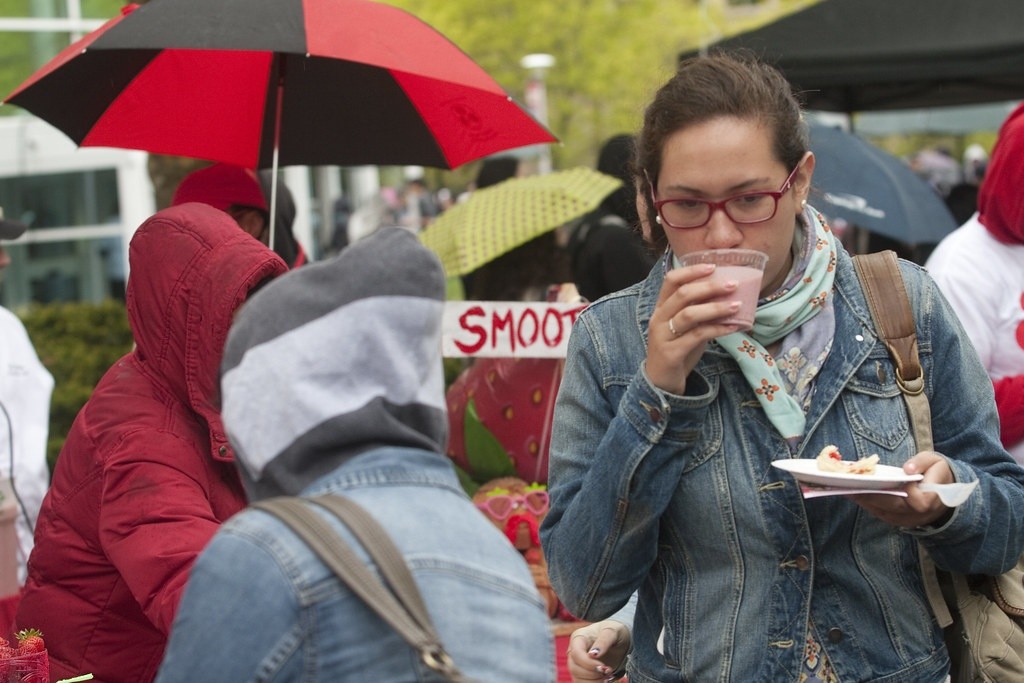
[806,125,956,247]
[0,1,563,250]
[419,166,625,278]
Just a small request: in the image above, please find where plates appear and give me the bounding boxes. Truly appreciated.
[769,457,925,489]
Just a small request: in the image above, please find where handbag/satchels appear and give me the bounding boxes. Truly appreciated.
[852,247,1023,683]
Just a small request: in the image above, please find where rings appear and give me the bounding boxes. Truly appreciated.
[669,318,683,338]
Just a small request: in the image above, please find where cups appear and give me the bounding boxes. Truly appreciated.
[679,247,769,332]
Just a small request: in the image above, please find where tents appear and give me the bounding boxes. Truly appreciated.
[679,0,1024,136]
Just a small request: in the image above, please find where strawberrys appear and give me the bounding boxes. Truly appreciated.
[0,627,48,683]
[445,358,564,484]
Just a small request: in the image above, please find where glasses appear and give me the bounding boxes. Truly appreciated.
[643,160,801,228]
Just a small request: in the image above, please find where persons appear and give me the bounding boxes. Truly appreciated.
[536,58,1023,683]
[162,162,310,273]
[0,220,55,587]
[831,147,987,268]
[157,227,558,683]
[924,105,1024,464]
[567,589,638,683]
[315,134,661,303]
[16,202,291,683]
